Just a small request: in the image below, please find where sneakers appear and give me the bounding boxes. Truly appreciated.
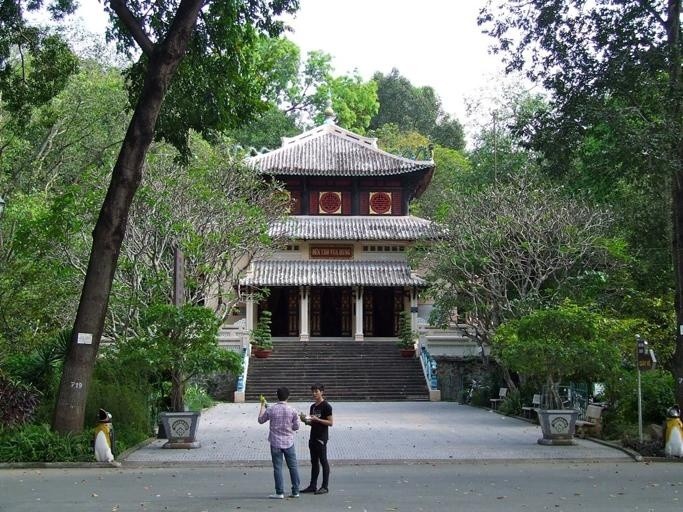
[269,494,284,499]
[289,493,300,498]
[314,486,328,494]
[300,485,317,493]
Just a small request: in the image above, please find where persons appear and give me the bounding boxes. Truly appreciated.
[257,387,300,499]
[299,383,333,495]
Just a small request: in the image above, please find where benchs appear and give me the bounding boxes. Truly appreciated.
[520,392,544,419]
[573,403,607,439]
[489,386,509,410]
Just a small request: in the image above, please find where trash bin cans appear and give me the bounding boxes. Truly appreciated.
[158,397,172,438]
[664,404,683,458]
[94,408,116,463]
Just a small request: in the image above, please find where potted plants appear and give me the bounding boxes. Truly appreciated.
[250,309,273,359]
[396,308,415,357]
[116,300,246,449]
[494,304,627,447]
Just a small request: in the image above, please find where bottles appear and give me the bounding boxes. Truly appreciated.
[259,395,268,409]
